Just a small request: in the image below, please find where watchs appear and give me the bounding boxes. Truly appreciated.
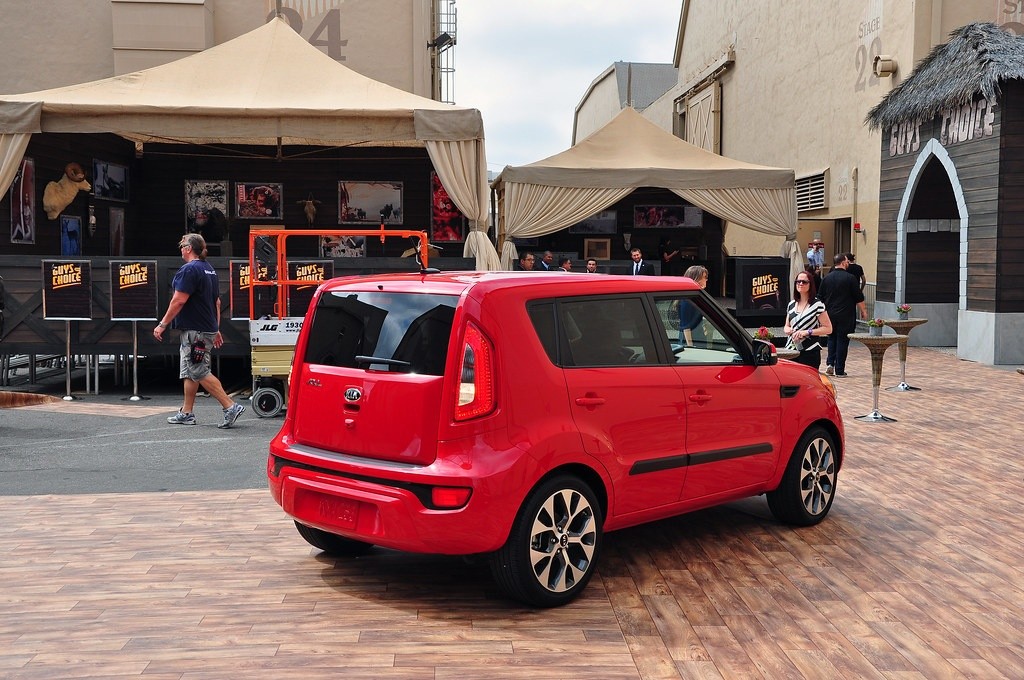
[807,328,812,336]
[159,322,166,328]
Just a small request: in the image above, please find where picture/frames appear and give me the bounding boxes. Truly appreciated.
[633,204,705,230]
[429,170,466,244]
[513,238,540,248]
[568,208,619,237]
[234,181,285,220]
[92,157,130,203]
[60,214,83,256]
[184,178,229,246]
[584,239,610,261]
[108,207,128,257]
[9,157,36,245]
[337,180,405,226]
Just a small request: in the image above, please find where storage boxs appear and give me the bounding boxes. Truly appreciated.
[248,315,304,347]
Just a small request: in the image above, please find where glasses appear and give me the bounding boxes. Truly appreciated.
[796,280,810,285]
[815,243,820,245]
[525,258,536,262]
[179,243,191,248]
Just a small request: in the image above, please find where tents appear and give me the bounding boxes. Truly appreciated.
[489,106,811,307]
[0,15,503,272]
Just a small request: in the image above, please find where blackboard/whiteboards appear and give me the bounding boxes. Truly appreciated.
[285,261,334,319]
[110,260,158,322]
[734,258,790,319]
[229,260,278,320]
[42,259,94,322]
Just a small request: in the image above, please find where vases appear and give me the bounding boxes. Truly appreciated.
[900,312,908,319]
[870,326,883,336]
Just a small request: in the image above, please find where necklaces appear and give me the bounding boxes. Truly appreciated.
[795,297,809,316]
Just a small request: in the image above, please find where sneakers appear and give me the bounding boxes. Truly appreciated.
[217,403,246,429]
[166,407,196,425]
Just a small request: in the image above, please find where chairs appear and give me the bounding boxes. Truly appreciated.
[580,320,622,363]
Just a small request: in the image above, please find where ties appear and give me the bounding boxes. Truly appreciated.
[635,263,639,275]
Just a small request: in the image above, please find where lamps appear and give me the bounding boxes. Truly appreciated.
[854,223,865,235]
[427,32,452,50]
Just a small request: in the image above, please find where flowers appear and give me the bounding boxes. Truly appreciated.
[897,304,912,314]
[869,317,884,328]
[752,326,775,342]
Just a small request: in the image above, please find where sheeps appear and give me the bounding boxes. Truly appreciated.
[43,162,92,221]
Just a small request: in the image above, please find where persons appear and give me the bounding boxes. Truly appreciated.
[616,232,638,260]
[153,234,247,429]
[678,265,714,350]
[783,271,833,370]
[805,238,868,378]
[514,248,657,276]
[661,237,682,276]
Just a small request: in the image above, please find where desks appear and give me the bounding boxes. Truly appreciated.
[883,317,929,392]
[726,348,800,360]
[846,332,910,423]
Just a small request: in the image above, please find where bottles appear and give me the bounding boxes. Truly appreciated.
[791,332,803,350]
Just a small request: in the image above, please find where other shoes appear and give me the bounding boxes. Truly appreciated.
[836,372,847,378]
[826,364,834,375]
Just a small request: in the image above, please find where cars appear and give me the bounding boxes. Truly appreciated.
[265,271,848,605]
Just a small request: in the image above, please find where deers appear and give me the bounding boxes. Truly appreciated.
[64,220,79,251]
